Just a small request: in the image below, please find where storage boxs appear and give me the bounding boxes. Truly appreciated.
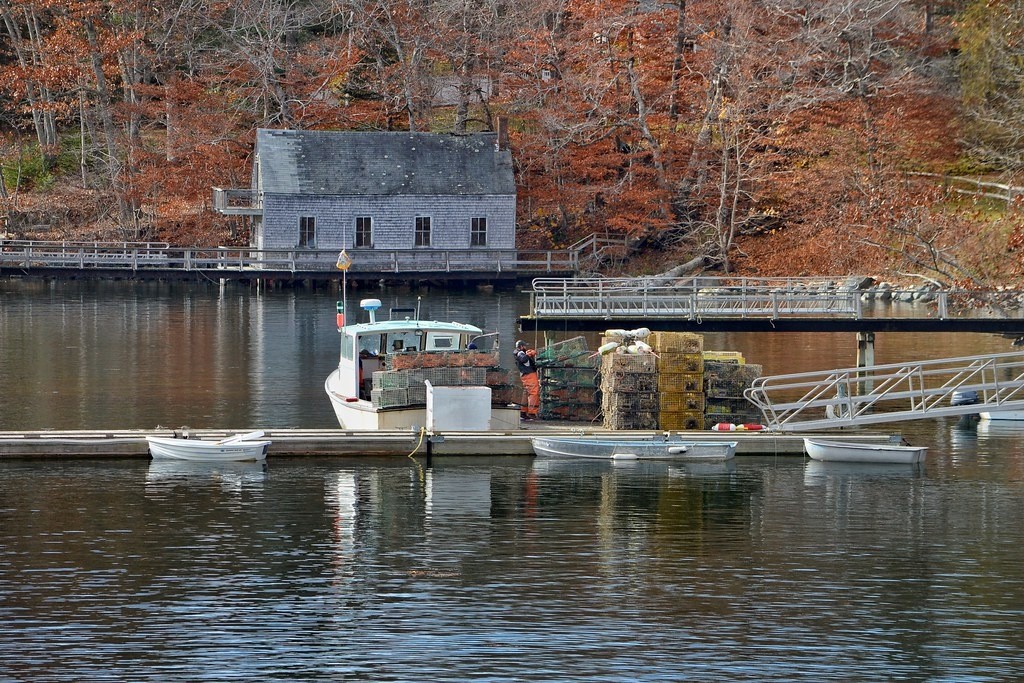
[372,348,499,389]
[426,385,492,429]
[531,331,762,430]
[371,388,408,409]
[408,386,427,405]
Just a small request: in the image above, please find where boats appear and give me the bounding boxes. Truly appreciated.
[803,437,929,463]
[950,389,1024,421]
[322,223,521,433]
[531,437,738,461]
[145,432,272,461]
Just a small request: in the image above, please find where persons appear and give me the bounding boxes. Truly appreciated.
[514,340,539,419]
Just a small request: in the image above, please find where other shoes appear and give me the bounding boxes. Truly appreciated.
[528,414,542,421]
[520,411,529,419]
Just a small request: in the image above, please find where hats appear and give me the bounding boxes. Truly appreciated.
[467,343,478,349]
[516,340,530,348]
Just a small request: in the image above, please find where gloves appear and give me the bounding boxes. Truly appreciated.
[528,350,538,358]
